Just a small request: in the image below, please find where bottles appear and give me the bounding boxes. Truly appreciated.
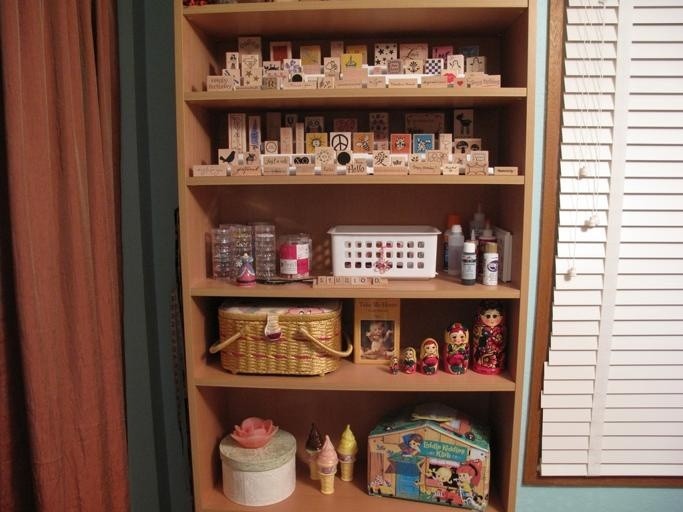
[443,201,499,286]
[210,222,312,287]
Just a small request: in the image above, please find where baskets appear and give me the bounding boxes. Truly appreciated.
[325,222,441,280]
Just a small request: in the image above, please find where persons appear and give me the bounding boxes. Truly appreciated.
[359,319,395,362]
[234,250,257,286]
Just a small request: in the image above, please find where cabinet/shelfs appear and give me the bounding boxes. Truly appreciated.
[172,1,534,507]
